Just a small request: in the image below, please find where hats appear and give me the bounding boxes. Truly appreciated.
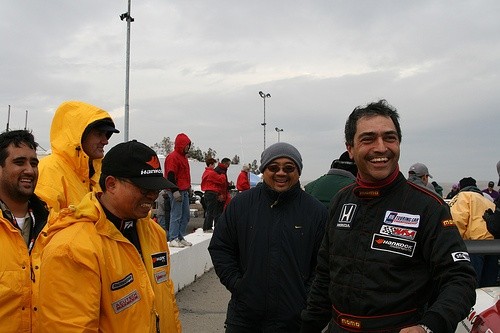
[91,120,121,134]
[101,140,179,191]
[331,151,358,177]
[452,184,457,188]
[488,181,494,187]
[258,142,303,174]
[408,163,433,179]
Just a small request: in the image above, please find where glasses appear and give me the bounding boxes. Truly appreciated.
[118,178,163,196]
[267,163,297,174]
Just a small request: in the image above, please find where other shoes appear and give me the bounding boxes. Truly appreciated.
[203,229,215,233]
[180,238,192,246]
[169,237,185,248]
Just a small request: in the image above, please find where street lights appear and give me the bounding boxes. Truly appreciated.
[258,90,272,150]
[275,127,284,142]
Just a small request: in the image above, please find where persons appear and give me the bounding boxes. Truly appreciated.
[201,157,250,233]
[34,101,120,212]
[207,142,330,333]
[0,128,58,333]
[39,139,183,333]
[154,188,173,230]
[164,134,193,247]
[295,99,477,333]
[303,150,359,213]
[406,159,499,288]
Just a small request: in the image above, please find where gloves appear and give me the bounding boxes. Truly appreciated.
[482,208,493,222]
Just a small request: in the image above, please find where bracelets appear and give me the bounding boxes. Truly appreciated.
[420,325,433,333]
